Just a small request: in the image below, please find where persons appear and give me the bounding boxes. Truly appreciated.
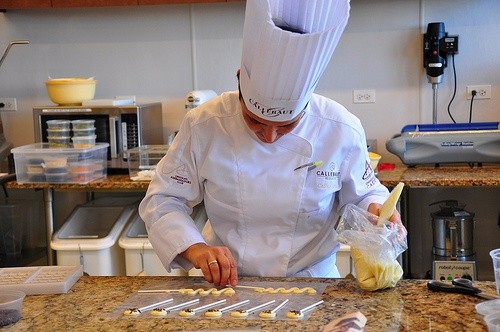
[136,0,406,291]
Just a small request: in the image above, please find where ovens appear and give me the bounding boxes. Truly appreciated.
[32,99,165,175]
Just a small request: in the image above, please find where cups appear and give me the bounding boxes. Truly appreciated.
[489,248,500,295]
[484,314,500,332]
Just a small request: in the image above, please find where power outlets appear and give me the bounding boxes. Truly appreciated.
[353,89,375,103]
[466,84,492,100]
[0,97,17,111]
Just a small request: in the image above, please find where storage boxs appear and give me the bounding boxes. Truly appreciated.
[51,195,209,276]
[11,142,109,185]
[127,145,171,181]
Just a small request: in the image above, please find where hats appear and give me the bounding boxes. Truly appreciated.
[239,0,350,123]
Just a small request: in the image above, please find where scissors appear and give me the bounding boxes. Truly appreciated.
[427,278,499,301]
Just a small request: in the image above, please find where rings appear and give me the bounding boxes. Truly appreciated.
[209,261,218,265]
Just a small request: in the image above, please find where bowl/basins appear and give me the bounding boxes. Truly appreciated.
[45,77,98,106]
